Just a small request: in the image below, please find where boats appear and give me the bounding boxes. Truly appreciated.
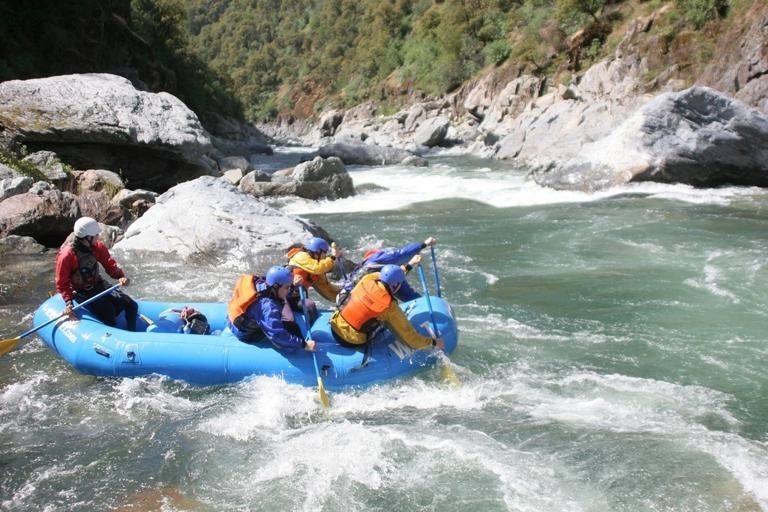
[31,292,458,389]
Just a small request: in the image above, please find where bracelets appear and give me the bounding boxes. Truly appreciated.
[433,339,436,346]
[404,263,413,271]
[330,256,337,261]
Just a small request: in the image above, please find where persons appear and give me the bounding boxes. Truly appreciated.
[225,266,316,354]
[160,306,210,335]
[55,217,137,331]
[284,238,343,327]
[345,237,436,302]
[330,263,444,350]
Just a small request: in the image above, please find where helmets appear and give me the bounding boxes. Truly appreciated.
[266,266,291,287]
[73,217,101,238]
[308,238,329,251]
[380,264,404,287]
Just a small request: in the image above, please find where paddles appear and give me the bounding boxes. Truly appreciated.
[299,287,330,409]
[0,283,122,358]
[417,262,462,389]
